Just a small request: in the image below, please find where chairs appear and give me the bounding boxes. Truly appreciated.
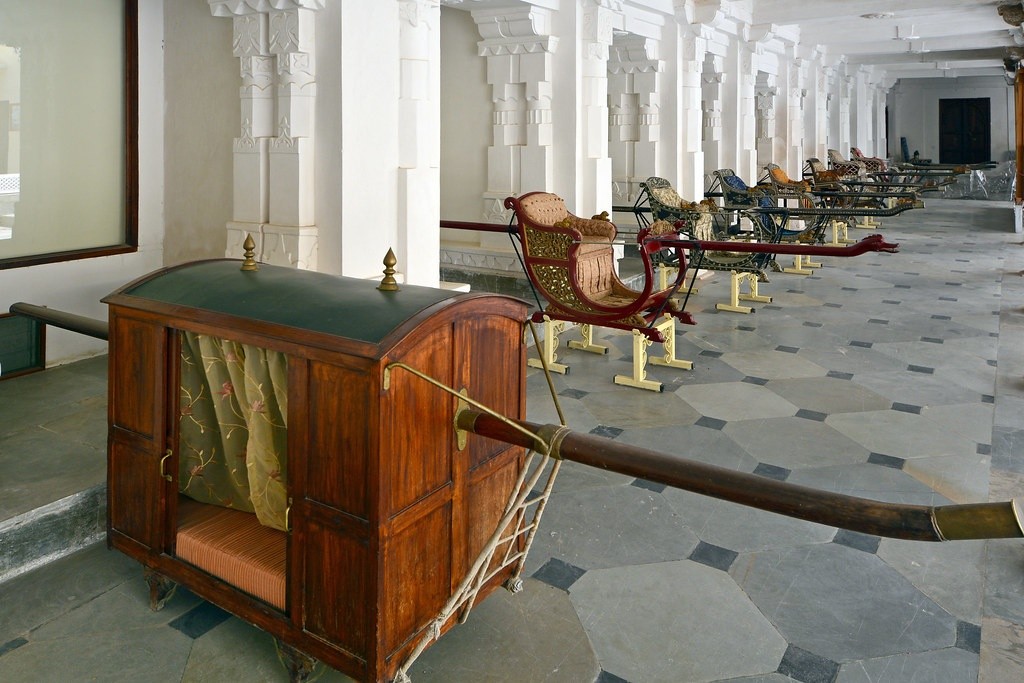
[504,146,916,393]
